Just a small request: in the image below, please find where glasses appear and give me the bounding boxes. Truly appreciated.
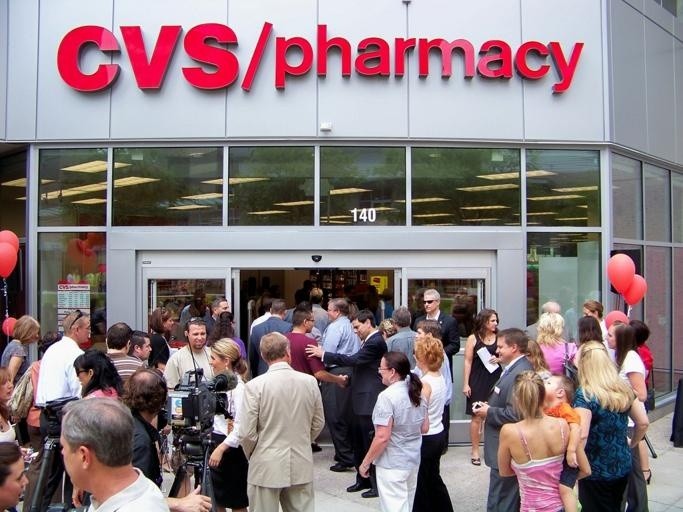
[423,300,437,304]
[77,367,89,376]
[186,317,207,327]
[70,310,83,329]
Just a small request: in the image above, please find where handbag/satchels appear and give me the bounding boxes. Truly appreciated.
[565,359,579,386]
[648,388,655,410]
[7,366,33,418]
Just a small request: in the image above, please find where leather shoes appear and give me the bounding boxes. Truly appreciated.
[347,482,379,497]
[330,462,354,471]
[311,443,322,451]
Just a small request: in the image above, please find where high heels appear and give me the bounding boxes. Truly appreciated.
[643,469,651,484]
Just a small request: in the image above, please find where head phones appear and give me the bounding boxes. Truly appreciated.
[148,368,168,416]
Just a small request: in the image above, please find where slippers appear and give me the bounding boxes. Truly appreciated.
[472,458,481,465]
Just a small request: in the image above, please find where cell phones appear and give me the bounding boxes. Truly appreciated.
[473,402,482,410]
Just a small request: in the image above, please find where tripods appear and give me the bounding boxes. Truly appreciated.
[168,461,218,512]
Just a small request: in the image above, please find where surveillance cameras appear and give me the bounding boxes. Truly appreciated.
[311,256,323,262]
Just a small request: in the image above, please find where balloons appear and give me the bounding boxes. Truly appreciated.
[607,253,635,295]
[0,243,18,279]
[0,230,20,252]
[59,232,106,284]
[621,273,647,305]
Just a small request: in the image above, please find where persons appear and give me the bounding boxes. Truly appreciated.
[2,280,653,512]
